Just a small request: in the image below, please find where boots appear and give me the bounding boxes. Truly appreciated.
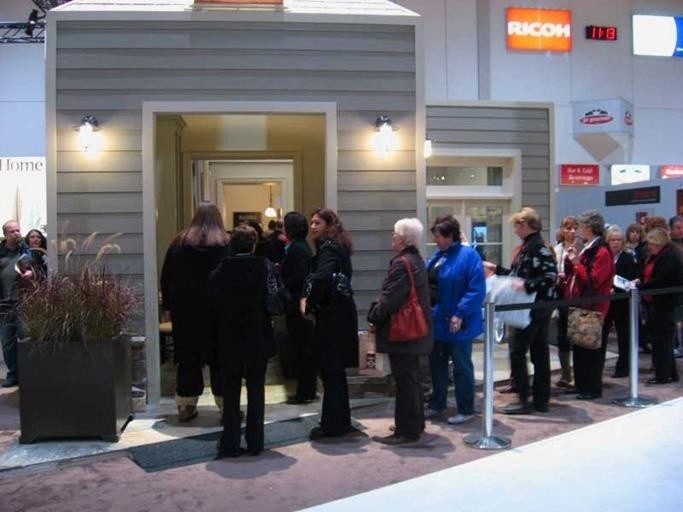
[175,395,198,421]
[215,396,244,424]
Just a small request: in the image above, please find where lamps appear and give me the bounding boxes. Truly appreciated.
[22,9,45,37]
[73,116,99,150]
[264,183,277,218]
[373,115,399,161]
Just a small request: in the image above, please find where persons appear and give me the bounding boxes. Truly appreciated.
[25,229,48,267]
[0,219,48,387]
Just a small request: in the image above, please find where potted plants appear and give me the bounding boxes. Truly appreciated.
[14,217,136,445]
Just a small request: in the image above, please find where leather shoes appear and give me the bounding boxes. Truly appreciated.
[558,374,602,399]
[499,382,517,393]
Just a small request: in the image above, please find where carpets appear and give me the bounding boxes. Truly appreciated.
[124,410,368,471]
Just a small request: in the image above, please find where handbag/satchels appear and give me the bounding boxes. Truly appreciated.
[483,274,537,328]
[547,306,604,349]
[389,287,428,342]
[359,330,392,378]
[305,273,354,316]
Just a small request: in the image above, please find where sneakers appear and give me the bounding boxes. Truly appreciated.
[447,413,475,423]
[498,401,530,414]
[532,403,547,411]
[381,435,415,444]
[422,407,447,417]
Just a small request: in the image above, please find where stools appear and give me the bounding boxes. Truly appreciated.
[157,320,175,365]
